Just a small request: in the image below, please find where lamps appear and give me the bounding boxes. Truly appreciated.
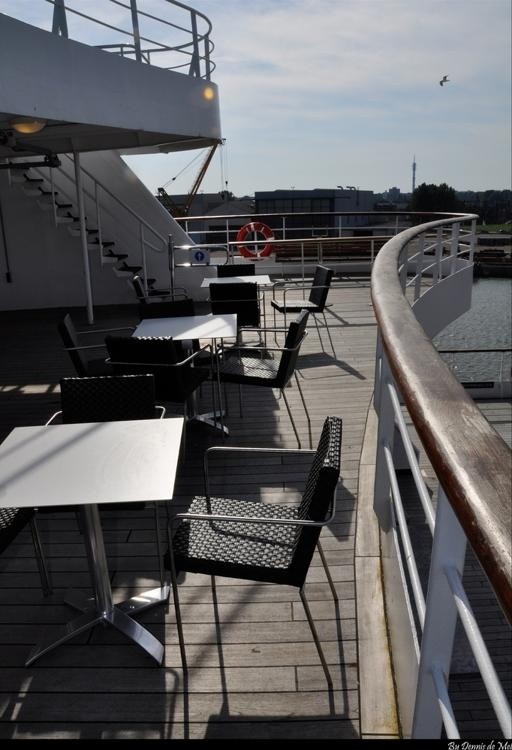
[9,117,47,134]
